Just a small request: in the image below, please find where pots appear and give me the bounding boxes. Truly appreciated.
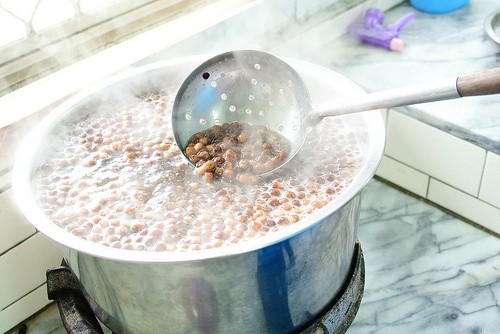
[13,53,388,333]
